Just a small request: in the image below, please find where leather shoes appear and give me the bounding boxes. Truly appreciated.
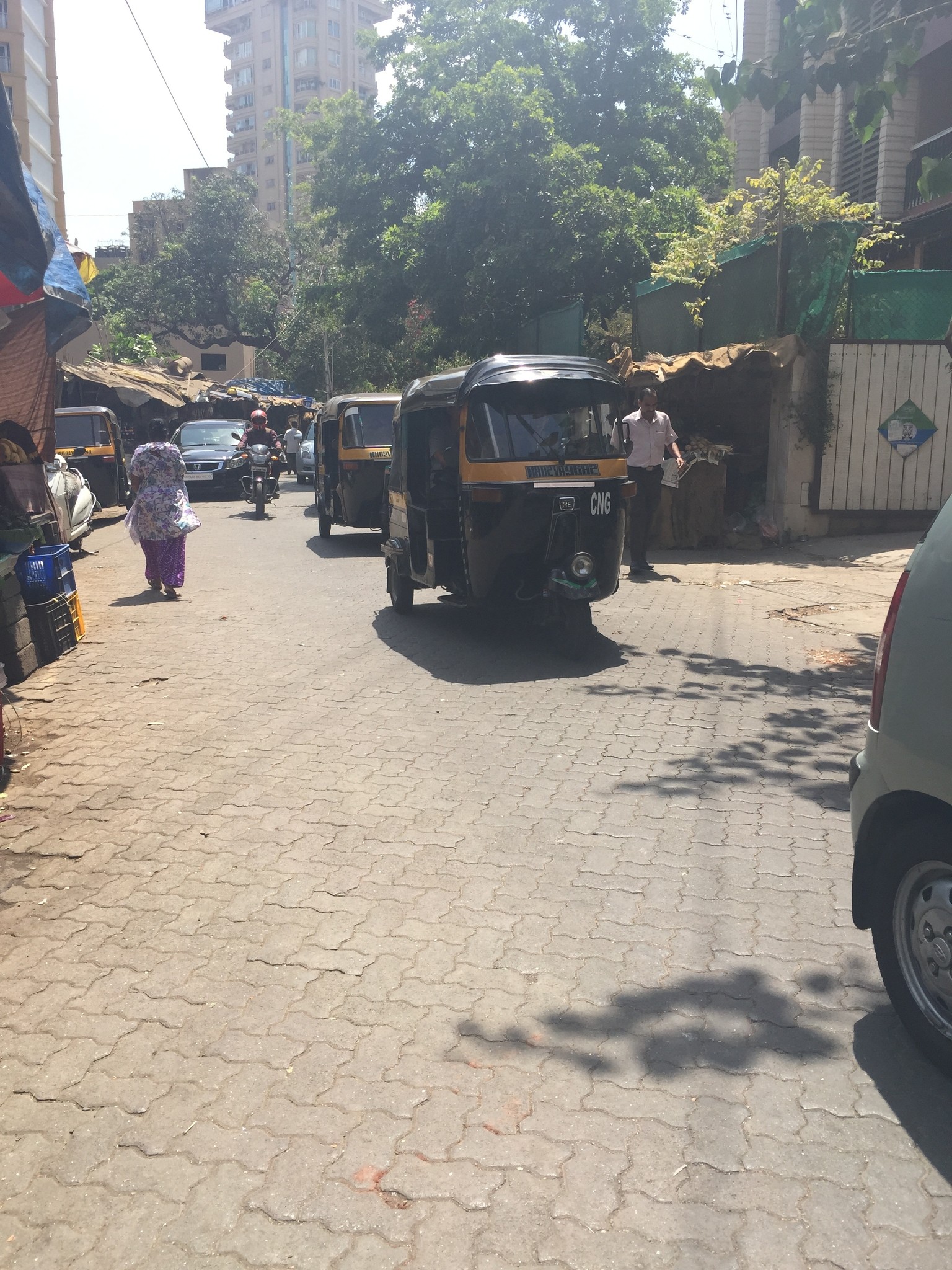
[640,561,655,570]
[631,565,648,573]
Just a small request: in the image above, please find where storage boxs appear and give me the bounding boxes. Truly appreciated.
[64,588,85,643]
[24,592,78,662]
[15,542,77,596]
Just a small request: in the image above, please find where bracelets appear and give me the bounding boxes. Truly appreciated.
[676,455,684,460]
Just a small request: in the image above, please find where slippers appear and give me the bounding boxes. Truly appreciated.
[146,578,162,591]
[164,591,181,598]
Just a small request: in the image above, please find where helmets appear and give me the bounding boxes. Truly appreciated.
[251,409,268,429]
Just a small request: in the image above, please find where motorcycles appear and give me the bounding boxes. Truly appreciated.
[314,393,402,538]
[380,355,636,662]
[231,433,284,520]
[54,406,128,509]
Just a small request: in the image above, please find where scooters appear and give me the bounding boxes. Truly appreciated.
[43,447,102,557]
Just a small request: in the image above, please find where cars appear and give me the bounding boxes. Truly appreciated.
[168,418,254,500]
[296,419,314,485]
[848,492,952,1079]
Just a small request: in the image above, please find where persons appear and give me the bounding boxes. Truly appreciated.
[236,408,282,500]
[611,385,685,575]
[283,420,303,475]
[128,419,194,599]
